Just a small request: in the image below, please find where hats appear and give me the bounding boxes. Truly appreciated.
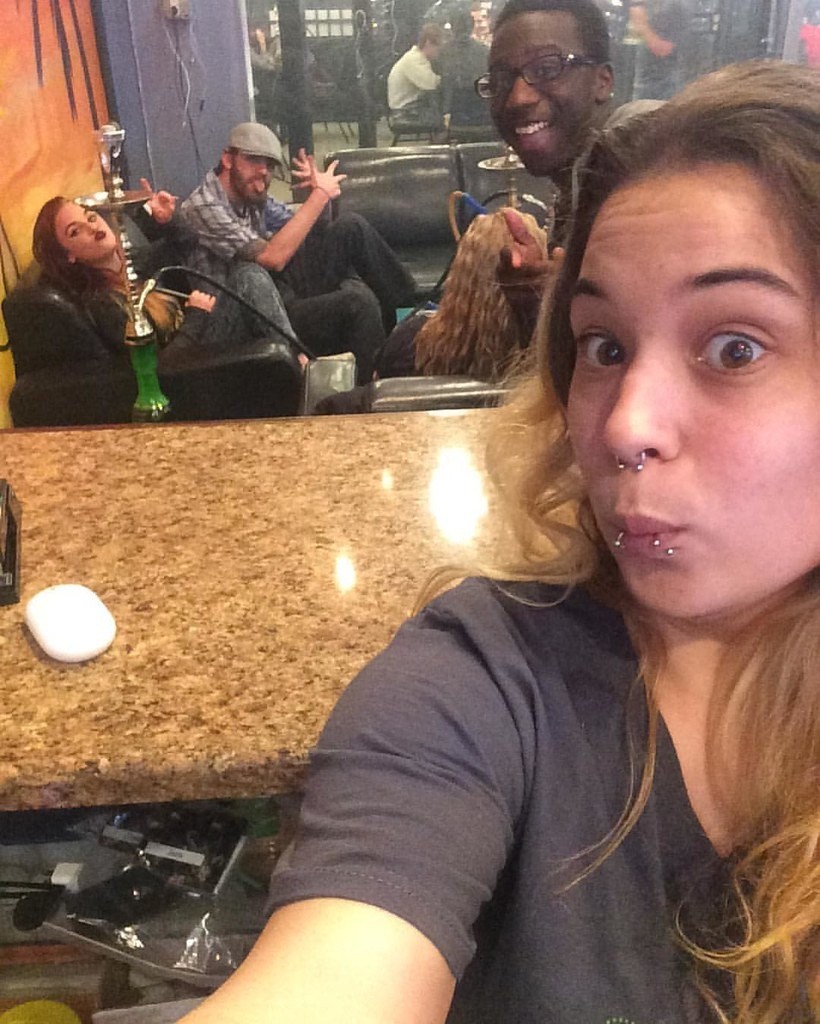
[228,122,284,167]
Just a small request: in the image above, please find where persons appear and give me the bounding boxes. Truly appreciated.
[0,0,820,431]
[167,54,820,1024]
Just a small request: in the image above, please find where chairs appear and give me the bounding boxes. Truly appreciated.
[319,73,559,299]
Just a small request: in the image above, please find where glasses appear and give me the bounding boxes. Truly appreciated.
[474,52,598,99]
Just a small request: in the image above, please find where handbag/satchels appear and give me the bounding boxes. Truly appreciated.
[388,105,445,132]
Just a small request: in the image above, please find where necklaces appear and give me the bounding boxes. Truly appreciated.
[233,201,247,217]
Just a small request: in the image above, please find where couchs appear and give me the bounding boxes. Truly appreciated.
[1,211,308,425]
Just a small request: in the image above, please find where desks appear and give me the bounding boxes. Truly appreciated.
[1,408,590,807]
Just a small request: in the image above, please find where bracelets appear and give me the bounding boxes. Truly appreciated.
[313,186,331,200]
